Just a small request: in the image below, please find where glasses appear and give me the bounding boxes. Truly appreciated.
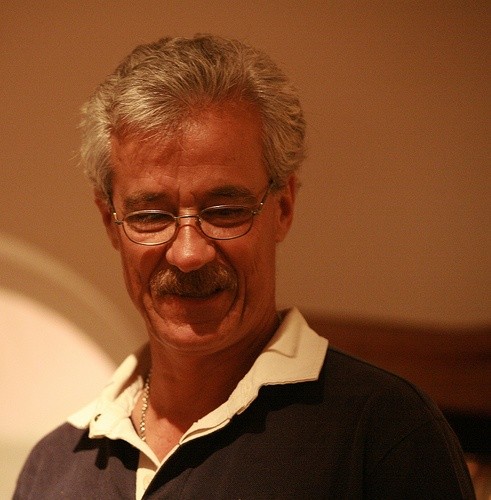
[106,179,276,247]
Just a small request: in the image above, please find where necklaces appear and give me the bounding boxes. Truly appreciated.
[139,369,152,441]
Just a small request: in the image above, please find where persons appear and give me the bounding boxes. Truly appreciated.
[9,35,478,500]
